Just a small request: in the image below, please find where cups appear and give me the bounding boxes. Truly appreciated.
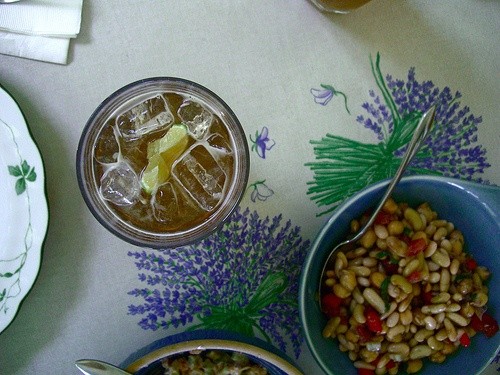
[76,77,249,251]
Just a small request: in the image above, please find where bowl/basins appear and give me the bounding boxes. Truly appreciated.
[120,330,303,375]
[299,176,499,375]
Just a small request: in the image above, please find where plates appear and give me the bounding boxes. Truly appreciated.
[0,88,50,337]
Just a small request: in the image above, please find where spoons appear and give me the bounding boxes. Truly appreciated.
[316,105,437,309]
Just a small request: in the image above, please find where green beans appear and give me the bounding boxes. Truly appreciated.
[321,197,498,375]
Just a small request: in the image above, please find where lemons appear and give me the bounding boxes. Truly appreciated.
[140,122,189,194]
